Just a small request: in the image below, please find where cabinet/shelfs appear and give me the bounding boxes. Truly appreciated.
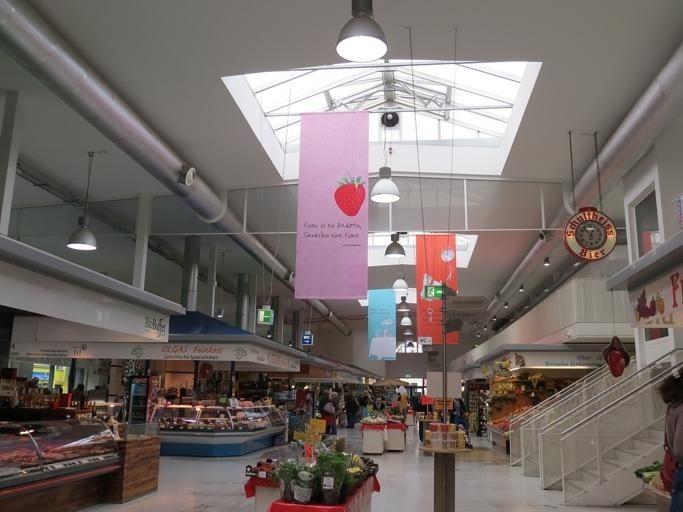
[0,417,162,512]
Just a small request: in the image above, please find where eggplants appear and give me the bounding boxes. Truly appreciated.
[649,296,656,317]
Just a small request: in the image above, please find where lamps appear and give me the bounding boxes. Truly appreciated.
[371,114,400,204]
[543,257,549,267]
[383,231,406,259]
[602,286,630,375]
[67,151,97,251]
[336,0,389,63]
[392,276,415,348]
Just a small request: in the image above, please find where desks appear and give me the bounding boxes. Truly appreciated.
[361,423,405,456]
[249,450,373,512]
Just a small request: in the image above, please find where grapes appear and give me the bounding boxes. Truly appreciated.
[636,289,649,320]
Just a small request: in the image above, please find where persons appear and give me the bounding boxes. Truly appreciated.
[177,388,186,416]
[660,375,682,511]
[319,390,384,436]
[457,424,470,444]
[23,376,85,409]
[448,398,469,423]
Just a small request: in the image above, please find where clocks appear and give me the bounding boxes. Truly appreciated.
[563,207,616,261]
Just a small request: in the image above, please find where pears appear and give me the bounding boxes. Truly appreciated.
[633,303,640,321]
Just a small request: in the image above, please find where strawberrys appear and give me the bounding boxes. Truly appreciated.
[334,175,366,216]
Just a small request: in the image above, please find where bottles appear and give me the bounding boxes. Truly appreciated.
[113,420,119,439]
